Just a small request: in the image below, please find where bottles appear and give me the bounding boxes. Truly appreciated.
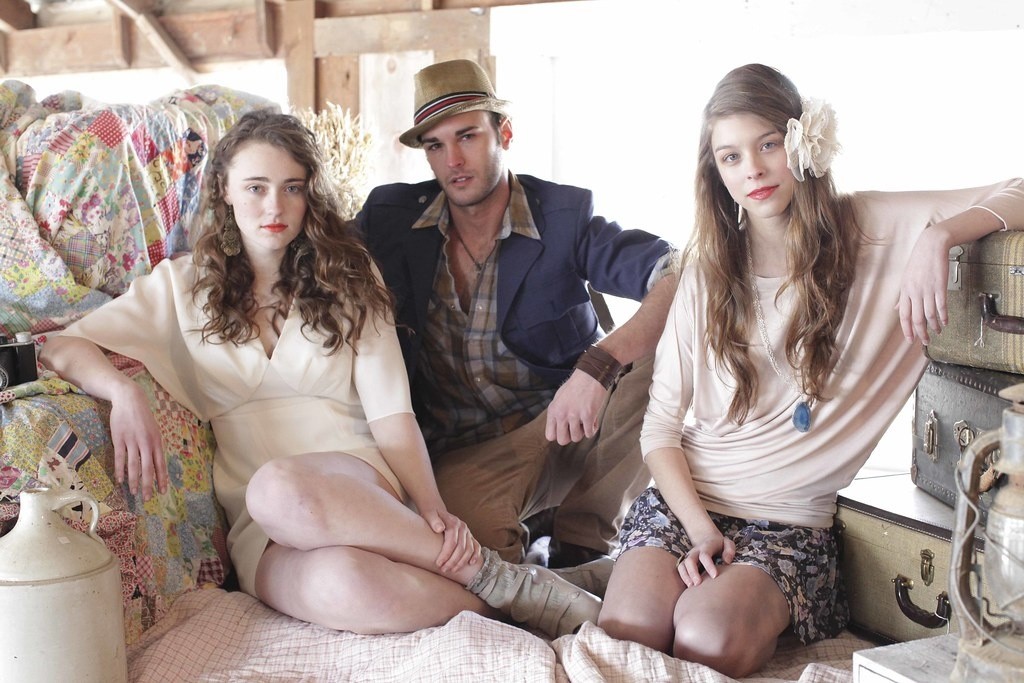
[0,487,130,683]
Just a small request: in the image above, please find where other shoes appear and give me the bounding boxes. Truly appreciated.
[562,557,616,598]
[545,539,602,568]
[501,562,602,640]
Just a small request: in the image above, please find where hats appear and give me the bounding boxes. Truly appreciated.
[398,60,516,149]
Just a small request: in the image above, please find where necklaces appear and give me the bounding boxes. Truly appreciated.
[451,211,482,274]
[744,225,812,433]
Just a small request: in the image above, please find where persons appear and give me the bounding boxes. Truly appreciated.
[344,60,681,571]
[597,63,1024,677]
[38,109,614,641]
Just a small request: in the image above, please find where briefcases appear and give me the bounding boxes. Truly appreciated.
[909,362,1024,524]
[834,472,989,646]
[922,226,1024,375]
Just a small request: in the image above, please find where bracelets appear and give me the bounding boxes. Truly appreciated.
[577,344,623,388]
